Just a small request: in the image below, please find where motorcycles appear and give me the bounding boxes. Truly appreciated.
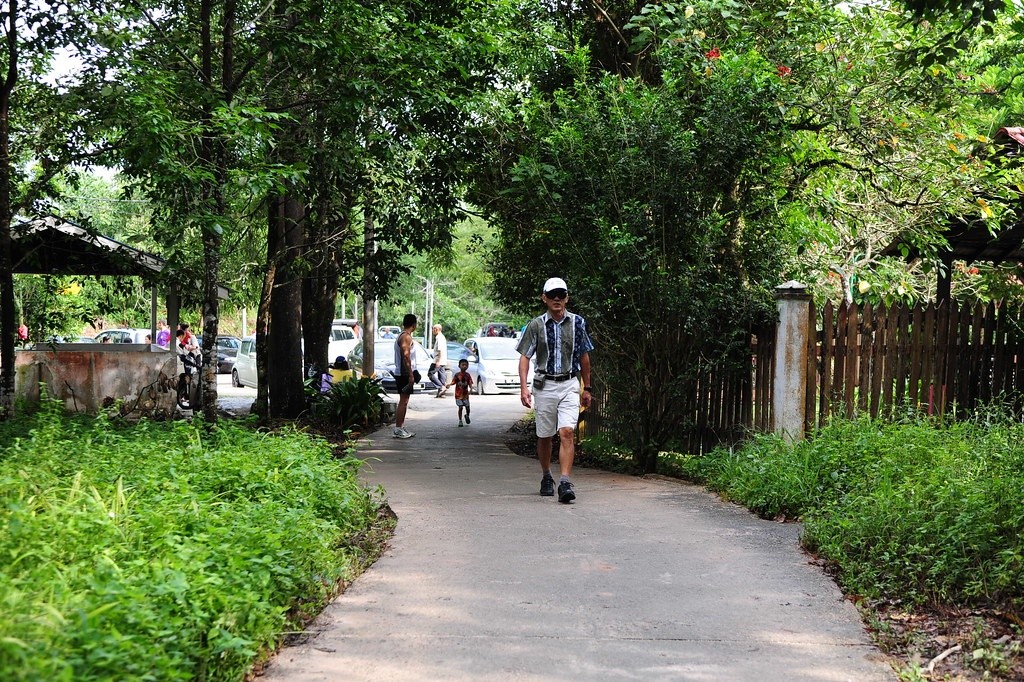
[177,354,202,411]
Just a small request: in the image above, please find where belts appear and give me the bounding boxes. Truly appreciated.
[544,373,575,380]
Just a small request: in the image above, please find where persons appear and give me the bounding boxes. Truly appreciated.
[446,359,473,427]
[392,314,417,440]
[427,324,447,398]
[124,338,132,343]
[487,326,515,337]
[329,356,356,384]
[145,319,202,373]
[103,336,111,343]
[515,277,594,502]
[379,327,397,339]
[353,323,359,338]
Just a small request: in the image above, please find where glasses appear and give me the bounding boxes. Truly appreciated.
[545,291,567,299]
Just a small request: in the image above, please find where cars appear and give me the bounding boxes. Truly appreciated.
[378,326,402,339]
[231,336,258,390]
[347,337,437,394]
[301,326,359,363]
[194,334,243,374]
[49,335,98,343]
[94,328,159,344]
[446,336,536,396]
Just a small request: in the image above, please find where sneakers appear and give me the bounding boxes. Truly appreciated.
[558,481,576,502]
[539,474,556,496]
[392,426,416,439]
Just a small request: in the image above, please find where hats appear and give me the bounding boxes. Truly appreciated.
[543,277,567,293]
[336,356,346,363]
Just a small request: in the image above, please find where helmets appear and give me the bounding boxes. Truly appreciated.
[181,322,189,330]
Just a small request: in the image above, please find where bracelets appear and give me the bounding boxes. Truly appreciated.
[584,387,591,392]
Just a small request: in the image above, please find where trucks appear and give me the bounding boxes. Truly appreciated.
[481,323,508,337]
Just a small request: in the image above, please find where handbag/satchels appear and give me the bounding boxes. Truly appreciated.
[321,373,334,397]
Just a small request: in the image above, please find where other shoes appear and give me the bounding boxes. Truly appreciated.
[464,414,470,424]
[436,386,446,397]
[458,421,463,427]
